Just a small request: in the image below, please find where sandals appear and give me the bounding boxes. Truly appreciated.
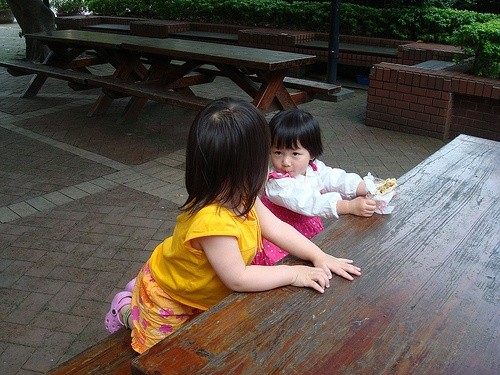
[105,277,137,334]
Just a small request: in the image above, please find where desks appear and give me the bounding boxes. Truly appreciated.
[132,133,500,375]
[20,29,318,121]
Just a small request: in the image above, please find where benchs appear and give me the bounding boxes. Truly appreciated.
[292,31,410,67]
[79,17,149,34]
[166,22,255,45]
[379,45,475,72]
[0,50,341,112]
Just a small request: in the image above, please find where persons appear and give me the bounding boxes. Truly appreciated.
[251,109,376,267]
[104,97,361,353]
